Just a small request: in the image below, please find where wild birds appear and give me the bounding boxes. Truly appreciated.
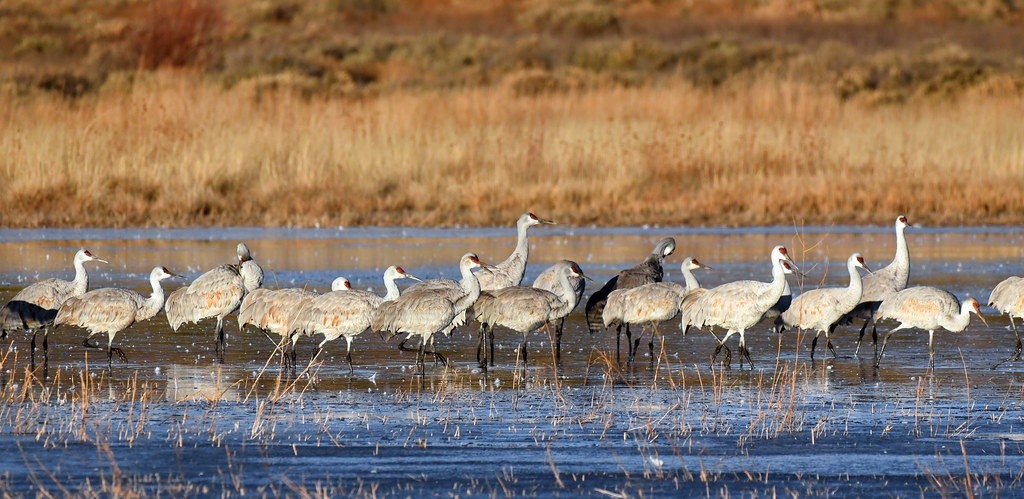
[678,246,808,371]
[602,257,714,372]
[285,266,423,373]
[464,260,595,374]
[52,266,184,366]
[584,236,677,365]
[166,243,264,352]
[371,252,502,374]
[986,276,1024,363]
[829,216,913,358]
[773,253,874,359]
[0,249,110,350]
[237,277,353,369]
[874,287,991,372]
[438,212,558,366]
